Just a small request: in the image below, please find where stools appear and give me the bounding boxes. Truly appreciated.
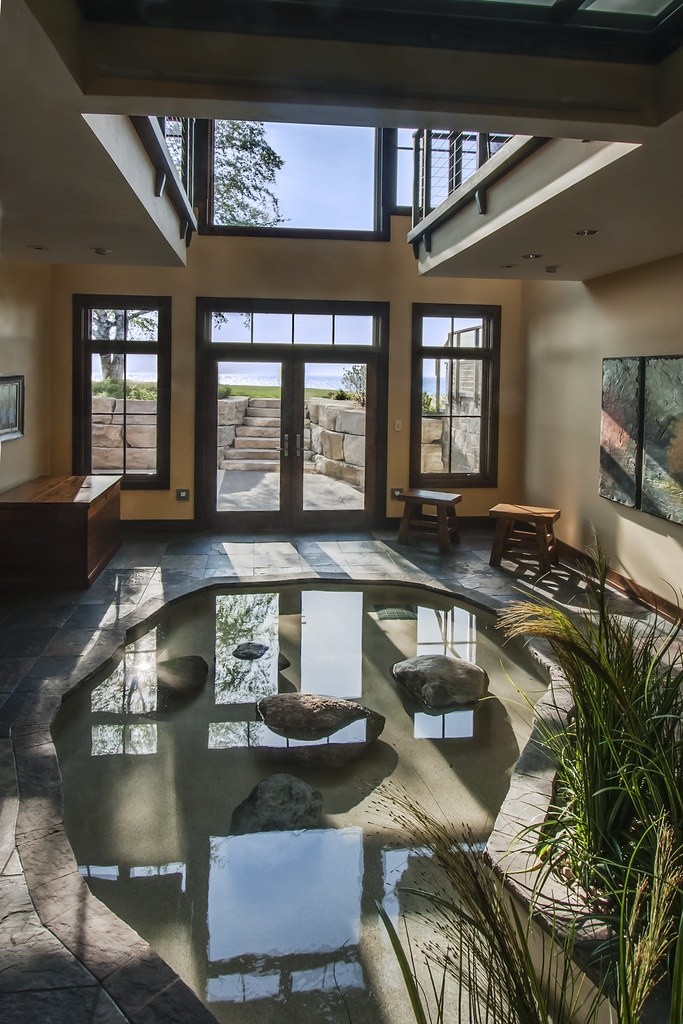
[487,502,562,576]
[396,489,463,551]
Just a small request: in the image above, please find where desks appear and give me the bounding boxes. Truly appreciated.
[0,475,124,590]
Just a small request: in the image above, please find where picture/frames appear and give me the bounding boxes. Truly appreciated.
[0,374,25,442]
